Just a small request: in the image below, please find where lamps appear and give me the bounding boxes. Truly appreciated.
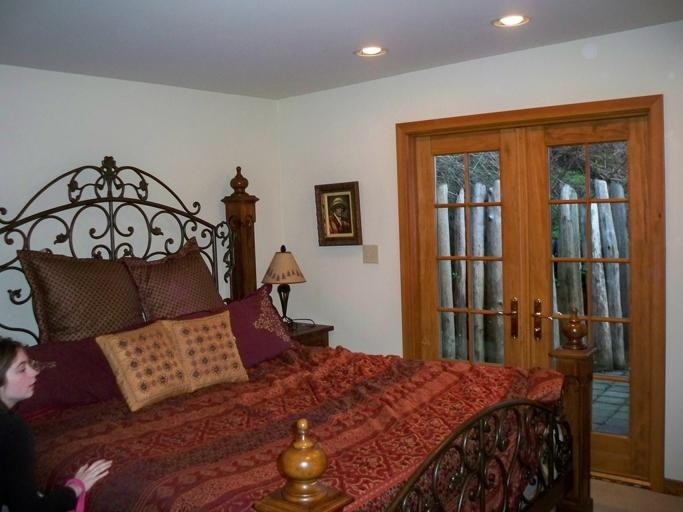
[261,242,308,327]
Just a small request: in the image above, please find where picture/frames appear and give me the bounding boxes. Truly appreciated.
[314,180,364,246]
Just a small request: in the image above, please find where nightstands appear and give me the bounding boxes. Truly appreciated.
[280,322,335,348]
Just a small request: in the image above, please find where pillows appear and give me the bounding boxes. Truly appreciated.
[119,238,227,324]
[13,322,154,414]
[14,249,146,344]
[156,311,249,392]
[95,320,191,413]
[163,284,293,369]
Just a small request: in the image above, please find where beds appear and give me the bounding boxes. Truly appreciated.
[0,157,597,512]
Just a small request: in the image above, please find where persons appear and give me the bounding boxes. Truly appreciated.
[0,336,113,512]
[329,198,350,233]
